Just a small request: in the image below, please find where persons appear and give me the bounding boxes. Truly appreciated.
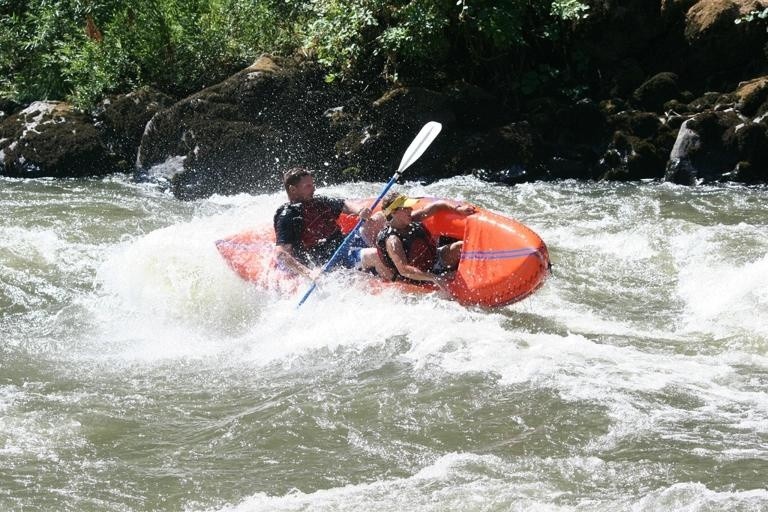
[376,191,475,293]
[273,167,394,282]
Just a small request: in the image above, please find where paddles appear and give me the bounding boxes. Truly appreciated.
[291,121,442,306]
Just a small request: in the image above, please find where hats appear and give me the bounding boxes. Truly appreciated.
[382,193,419,218]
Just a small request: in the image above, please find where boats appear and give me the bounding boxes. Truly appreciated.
[214,195,552,309]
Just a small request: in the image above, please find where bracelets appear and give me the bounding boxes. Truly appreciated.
[454,205,461,211]
